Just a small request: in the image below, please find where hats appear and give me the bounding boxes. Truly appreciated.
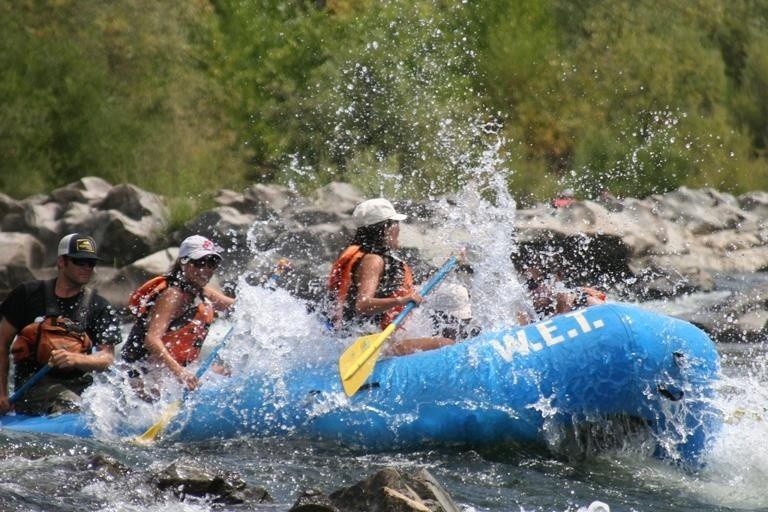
[352,198,408,229]
[178,235,224,261]
[58,233,103,260]
[435,284,473,320]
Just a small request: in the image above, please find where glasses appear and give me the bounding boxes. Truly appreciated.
[67,258,97,268]
[190,256,218,269]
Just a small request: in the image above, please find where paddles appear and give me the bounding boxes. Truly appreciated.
[137,259,289,444]
[340,246,468,398]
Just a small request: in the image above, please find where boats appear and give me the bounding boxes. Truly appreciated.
[0,304,724,474]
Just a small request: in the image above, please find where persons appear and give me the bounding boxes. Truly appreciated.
[325,197,456,355]
[0,231,122,422]
[516,246,606,325]
[428,283,480,342]
[117,234,238,405]
